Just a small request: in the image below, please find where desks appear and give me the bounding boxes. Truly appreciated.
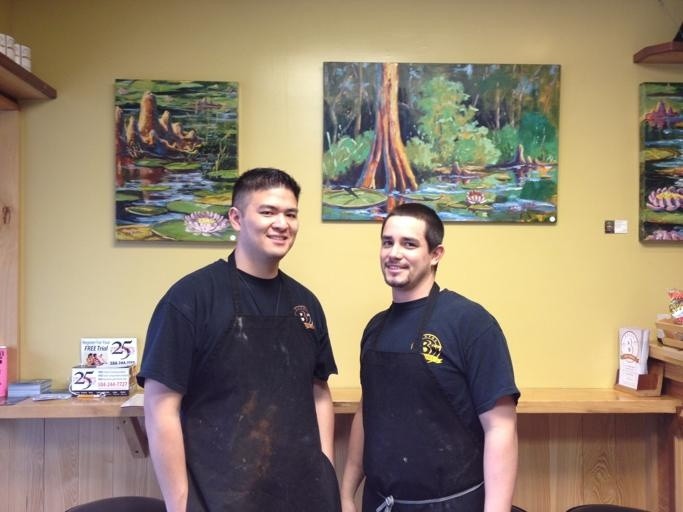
[0,386,682,511]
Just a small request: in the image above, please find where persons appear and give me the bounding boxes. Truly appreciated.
[139,167,347,510]
[339,203,524,511]
